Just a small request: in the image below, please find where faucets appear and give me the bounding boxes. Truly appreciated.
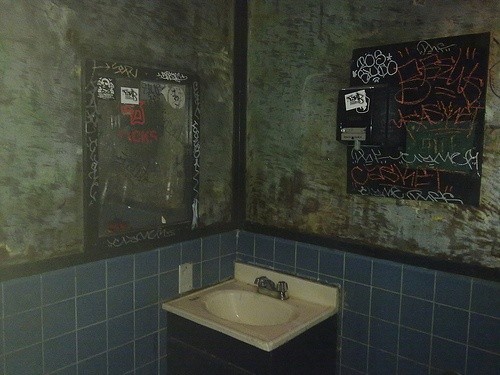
[258,278,277,297]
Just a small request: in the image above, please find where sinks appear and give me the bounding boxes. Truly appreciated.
[162,278,336,353]
